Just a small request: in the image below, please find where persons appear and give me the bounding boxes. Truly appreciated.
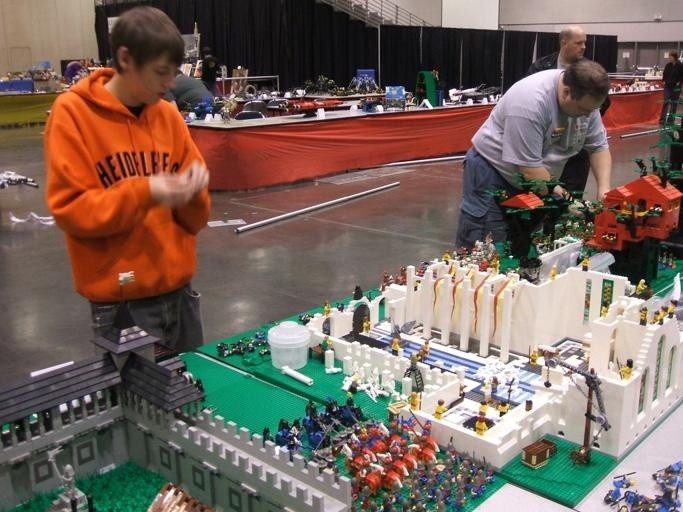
[201,46,219,96]
[454,60,612,253]
[165,69,214,110]
[658,50,683,126]
[41,9,212,360]
[527,25,611,200]
